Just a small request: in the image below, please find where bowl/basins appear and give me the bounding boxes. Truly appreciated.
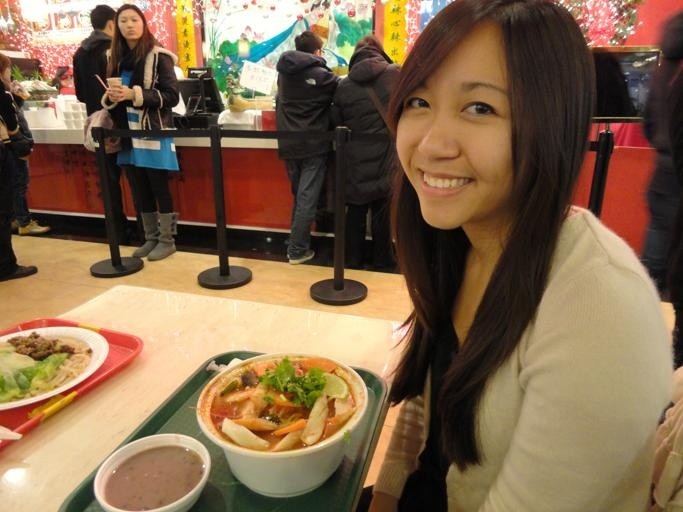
[27,90,58,101]
[91,432,212,511]
[195,350,369,500]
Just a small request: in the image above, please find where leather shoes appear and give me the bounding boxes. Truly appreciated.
[0,264,39,282]
[19,221,50,236]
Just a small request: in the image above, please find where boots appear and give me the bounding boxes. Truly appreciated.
[147,211,177,262]
[131,210,160,257]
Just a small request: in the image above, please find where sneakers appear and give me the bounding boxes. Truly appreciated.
[289,249,316,265]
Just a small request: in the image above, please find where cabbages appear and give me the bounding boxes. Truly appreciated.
[1,350,67,401]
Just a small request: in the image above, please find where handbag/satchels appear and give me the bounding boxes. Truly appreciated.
[82,49,123,154]
[364,83,396,134]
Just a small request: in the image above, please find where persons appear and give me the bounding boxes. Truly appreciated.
[369,0,675,512]
[72,5,147,243]
[640,4,683,371]
[331,38,405,272]
[0,54,55,236]
[272,32,344,267]
[0,73,39,281]
[102,4,183,261]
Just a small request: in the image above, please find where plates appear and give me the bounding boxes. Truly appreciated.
[60,349,388,512]
[1,317,143,455]
[1,325,110,413]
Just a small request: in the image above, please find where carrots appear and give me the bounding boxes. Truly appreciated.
[275,419,305,434]
[235,418,277,429]
[304,357,337,373]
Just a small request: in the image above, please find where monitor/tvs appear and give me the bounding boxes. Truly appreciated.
[174,78,225,115]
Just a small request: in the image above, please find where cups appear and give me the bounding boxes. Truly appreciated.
[81,111,88,119]
[63,111,71,119]
[72,111,81,120]
[64,120,73,129]
[81,103,86,111]
[71,104,80,111]
[74,120,83,128]
[107,78,122,103]
[83,119,88,126]
